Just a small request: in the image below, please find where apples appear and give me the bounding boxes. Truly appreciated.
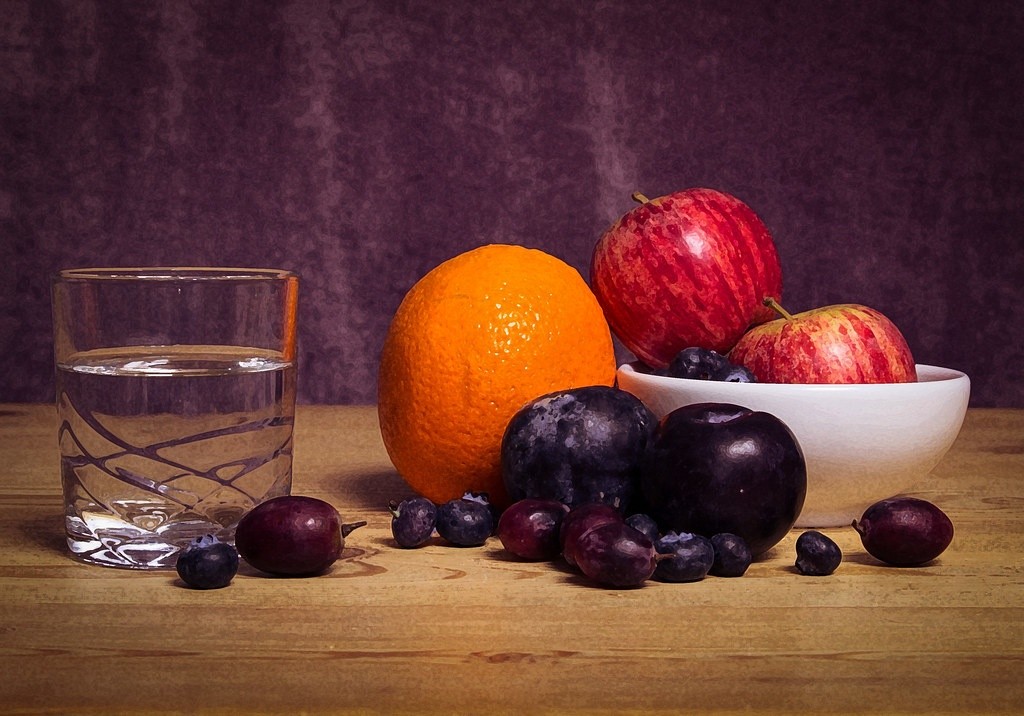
[727,295,917,385]
[591,186,784,367]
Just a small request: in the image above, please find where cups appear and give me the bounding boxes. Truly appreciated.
[51,267,299,572]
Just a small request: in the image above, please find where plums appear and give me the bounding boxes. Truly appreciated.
[501,387,660,519]
[641,403,807,552]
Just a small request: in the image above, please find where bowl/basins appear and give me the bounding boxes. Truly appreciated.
[616,364,972,528]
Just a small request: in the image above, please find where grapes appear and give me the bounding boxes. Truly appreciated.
[495,498,663,587]
[235,496,347,576]
[850,495,954,565]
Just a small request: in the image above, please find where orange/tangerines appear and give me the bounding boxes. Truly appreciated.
[377,243,614,504]
